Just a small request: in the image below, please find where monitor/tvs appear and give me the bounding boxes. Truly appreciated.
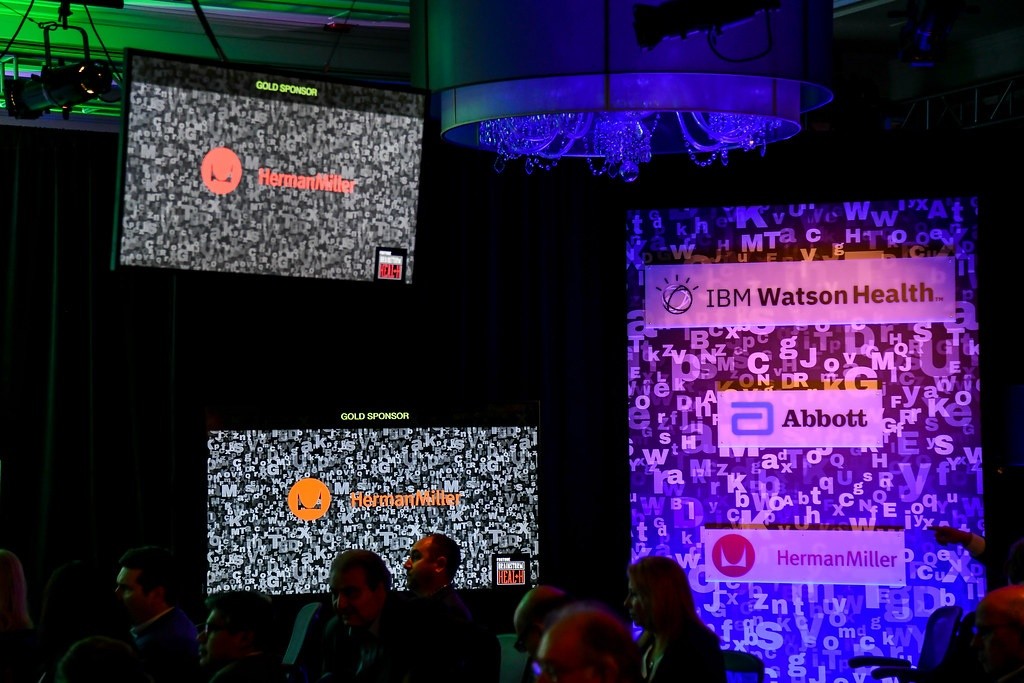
[186,406,546,619]
[106,44,434,296]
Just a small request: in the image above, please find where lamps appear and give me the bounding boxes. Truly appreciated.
[632,0,783,64]
[4,4,114,119]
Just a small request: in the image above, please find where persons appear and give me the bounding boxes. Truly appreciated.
[0,525,1024,683]
